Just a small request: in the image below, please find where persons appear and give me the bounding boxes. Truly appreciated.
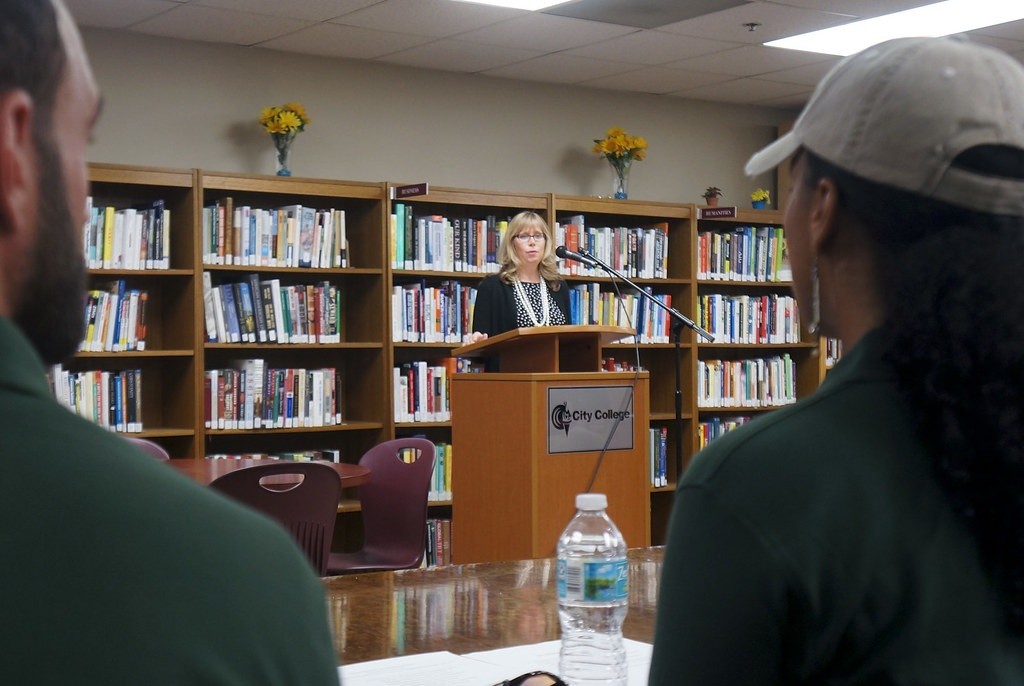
[462,211,571,347]
[0,0,343,685]
[649,33,1024,686]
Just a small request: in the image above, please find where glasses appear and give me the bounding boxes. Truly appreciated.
[495,670,565,686]
[514,233,544,242]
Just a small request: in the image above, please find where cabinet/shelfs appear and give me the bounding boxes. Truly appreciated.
[200,164,390,464]
[551,190,696,494]
[384,173,558,563]
[696,195,824,459]
[46,157,201,457]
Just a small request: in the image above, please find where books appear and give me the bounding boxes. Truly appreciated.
[394,358,484,423]
[202,271,340,344]
[556,214,669,279]
[81,195,170,270]
[391,203,510,273]
[203,196,349,268]
[698,353,797,408]
[392,279,477,342]
[697,293,801,343]
[420,519,453,570]
[697,228,783,282]
[697,417,750,452]
[389,575,490,654]
[626,560,661,610]
[602,358,634,372]
[827,339,841,364]
[397,442,452,501]
[207,450,339,479]
[78,280,148,351]
[46,364,142,433]
[204,358,342,429]
[651,426,668,487]
[567,283,671,344]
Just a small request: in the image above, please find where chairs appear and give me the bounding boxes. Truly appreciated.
[327,434,449,573]
[211,462,346,575]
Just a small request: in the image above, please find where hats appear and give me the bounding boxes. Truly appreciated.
[747,33,1023,217]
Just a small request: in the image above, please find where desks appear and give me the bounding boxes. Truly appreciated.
[322,546,666,686]
[161,453,373,503]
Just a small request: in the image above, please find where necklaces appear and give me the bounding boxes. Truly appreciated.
[513,272,549,327]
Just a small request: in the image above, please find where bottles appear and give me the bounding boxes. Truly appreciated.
[557,493,627,686]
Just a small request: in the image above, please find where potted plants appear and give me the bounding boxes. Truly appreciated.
[699,186,723,206]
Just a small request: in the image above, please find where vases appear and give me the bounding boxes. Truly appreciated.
[752,198,769,209]
[271,134,295,176]
[609,157,635,199]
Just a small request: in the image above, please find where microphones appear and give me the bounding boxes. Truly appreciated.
[556,246,605,271]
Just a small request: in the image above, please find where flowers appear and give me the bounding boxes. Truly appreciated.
[749,185,776,204]
[591,120,650,167]
[256,96,315,148]
